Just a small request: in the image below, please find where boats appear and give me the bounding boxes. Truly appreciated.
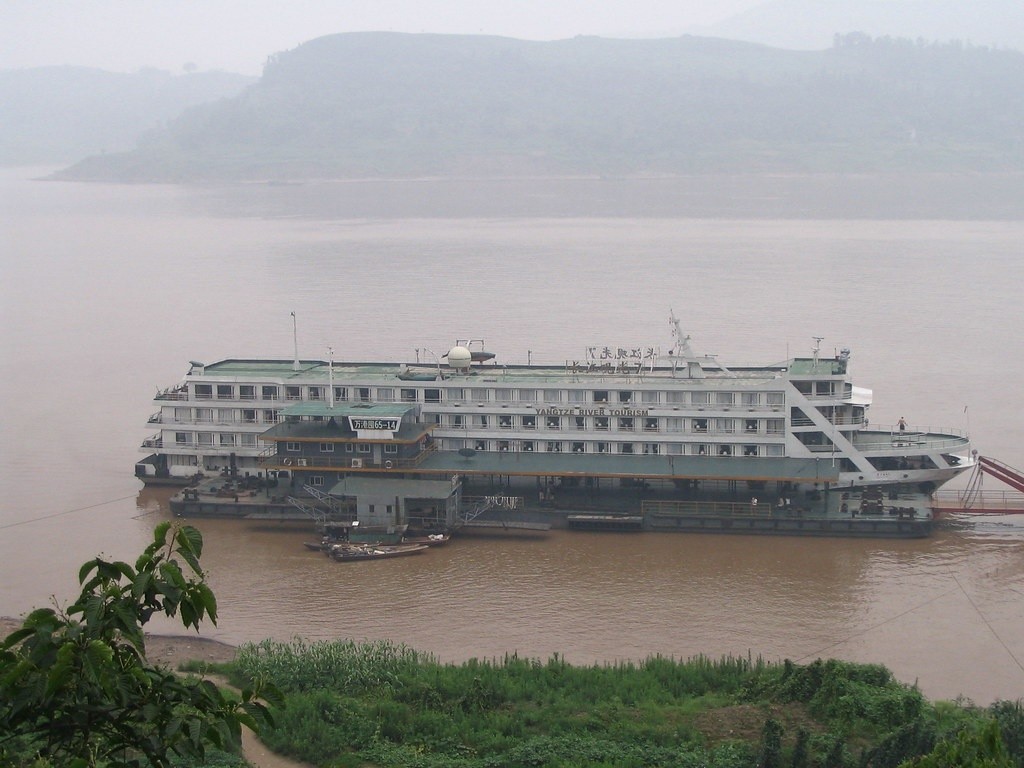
[327,545,429,561]
[304,524,451,551]
[134,308,981,496]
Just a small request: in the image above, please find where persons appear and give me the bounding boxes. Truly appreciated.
[750,496,758,515]
[895,416,908,436]
